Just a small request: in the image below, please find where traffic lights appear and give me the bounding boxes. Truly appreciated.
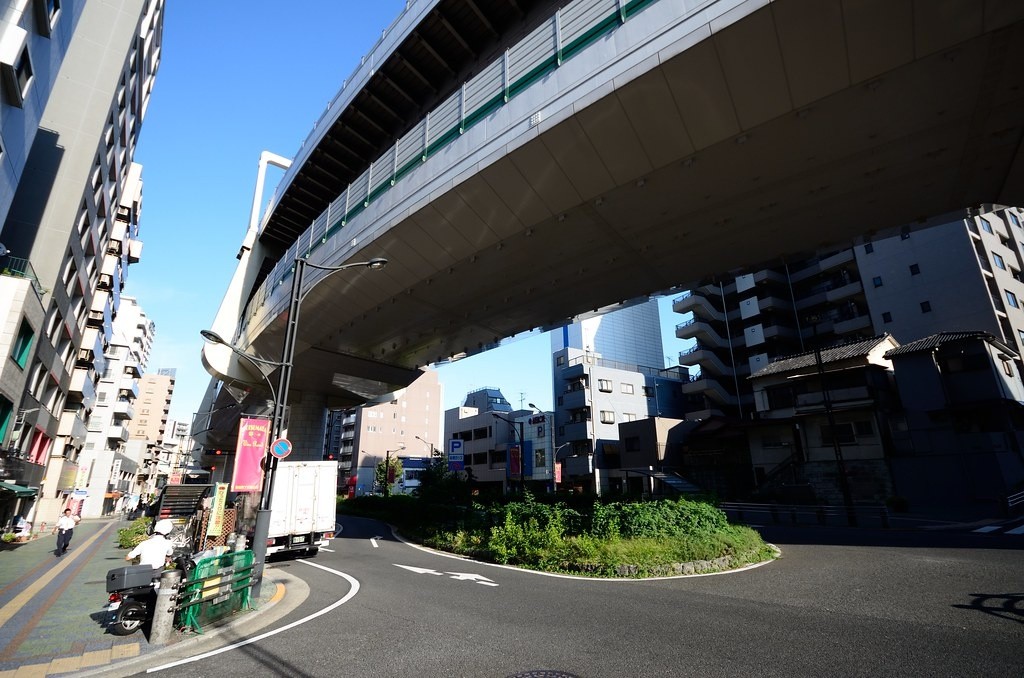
[328,453,334,460]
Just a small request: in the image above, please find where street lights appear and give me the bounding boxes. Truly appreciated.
[199,255,388,612]
[415,436,434,467]
[325,403,367,460]
[491,413,524,491]
[361,451,377,495]
[180,403,237,485]
[384,446,406,485]
[529,403,570,495]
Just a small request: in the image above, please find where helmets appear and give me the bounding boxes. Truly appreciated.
[154,519,173,536]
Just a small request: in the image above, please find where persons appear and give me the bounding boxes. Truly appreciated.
[126,519,174,569]
[52,508,79,557]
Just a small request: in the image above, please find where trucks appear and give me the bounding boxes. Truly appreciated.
[264,460,339,562]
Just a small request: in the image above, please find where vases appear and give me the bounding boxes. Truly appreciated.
[16,534,32,542]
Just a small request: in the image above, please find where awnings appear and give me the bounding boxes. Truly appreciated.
[0,482,35,497]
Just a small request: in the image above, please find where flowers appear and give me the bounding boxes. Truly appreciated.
[20,522,31,534]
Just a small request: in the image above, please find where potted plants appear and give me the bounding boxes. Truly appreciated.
[33,531,39,539]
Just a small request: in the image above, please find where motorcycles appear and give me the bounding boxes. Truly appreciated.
[105,549,197,636]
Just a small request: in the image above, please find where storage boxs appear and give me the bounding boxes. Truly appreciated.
[106,564,153,592]
